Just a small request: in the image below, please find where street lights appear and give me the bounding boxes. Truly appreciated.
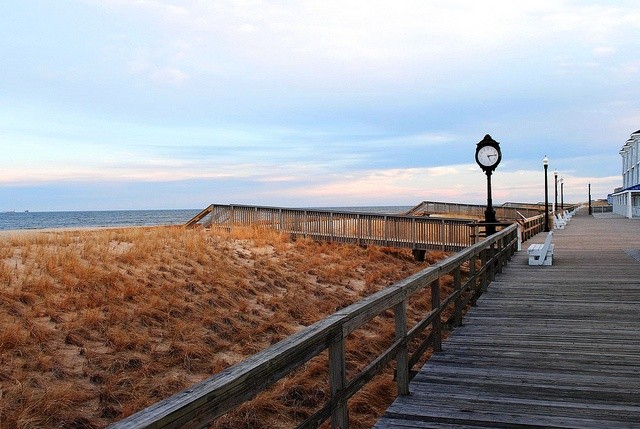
[561,176,563,209]
[542,155,551,232]
[554,168,557,211]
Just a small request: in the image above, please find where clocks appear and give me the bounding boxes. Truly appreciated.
[476,142,501,170]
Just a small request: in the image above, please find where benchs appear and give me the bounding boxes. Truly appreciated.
[553,207,576,230]
[527,231,555,267]
